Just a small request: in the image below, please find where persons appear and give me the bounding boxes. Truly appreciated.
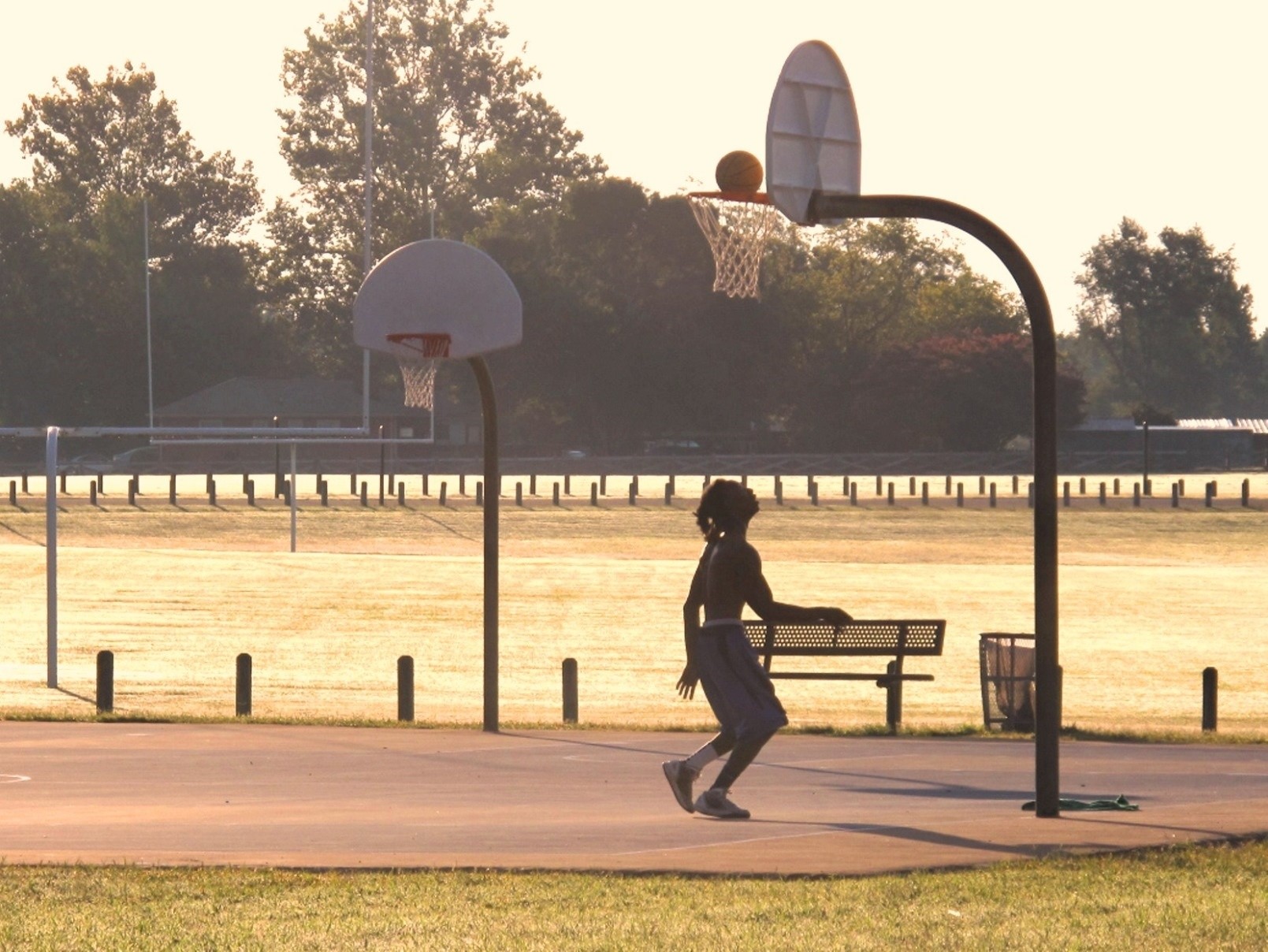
[659,479,856,817]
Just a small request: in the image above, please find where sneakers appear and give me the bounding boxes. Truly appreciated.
[693,790,750,818]
[661,760,697,814]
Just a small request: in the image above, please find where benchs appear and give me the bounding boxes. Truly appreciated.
[741,619,947,736]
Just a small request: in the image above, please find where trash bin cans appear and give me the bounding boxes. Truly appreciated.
[979,632,1036,735]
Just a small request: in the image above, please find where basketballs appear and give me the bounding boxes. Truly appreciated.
[716,151,763,192]
